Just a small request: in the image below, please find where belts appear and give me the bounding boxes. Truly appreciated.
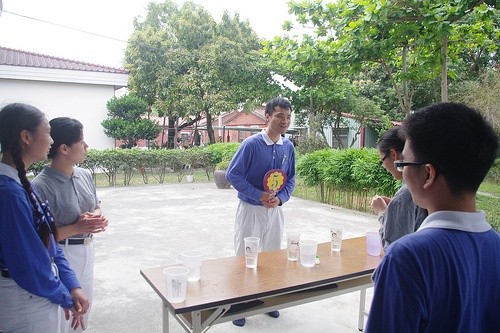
[57,237,91,245]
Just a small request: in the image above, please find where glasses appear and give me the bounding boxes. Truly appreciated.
[393,160,424,172]
[379,150,390,167]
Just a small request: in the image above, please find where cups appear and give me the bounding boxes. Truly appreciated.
[182,249,206,283]
[285,231,300,261]
[162,266,190,303]
[299,239,317,267]
[243,237,260,268]
[365,232,383,257]
[329,225,343,252]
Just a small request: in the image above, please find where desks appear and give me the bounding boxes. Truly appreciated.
[139,234,385,333]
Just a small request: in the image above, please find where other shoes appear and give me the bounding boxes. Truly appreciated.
[265,310,280,318]
[232,318,246,327]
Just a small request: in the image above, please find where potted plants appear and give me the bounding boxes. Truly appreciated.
[185,168,194,182]
[213,160,231,189]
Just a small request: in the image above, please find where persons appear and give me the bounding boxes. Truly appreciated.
[363,101,500,333]
[0,103,90,333]
[30,117,108,333]
[370,126,428,254]
[225,97,296,326]
